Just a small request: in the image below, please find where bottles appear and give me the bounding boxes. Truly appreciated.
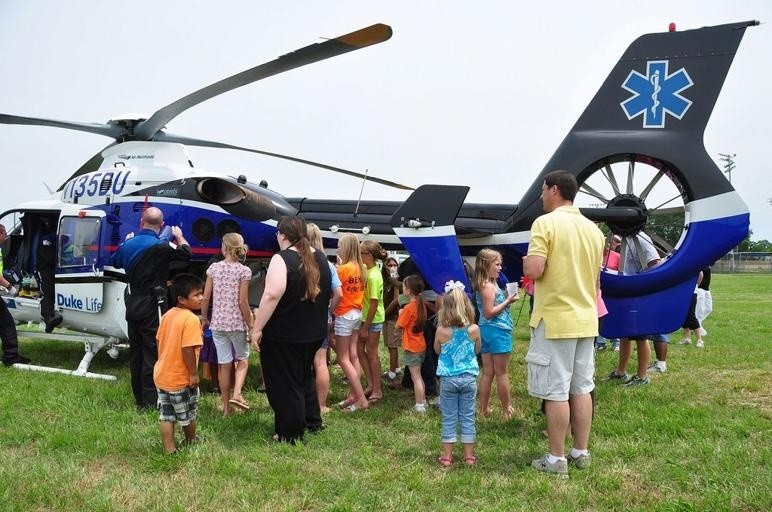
[203,329,212,340]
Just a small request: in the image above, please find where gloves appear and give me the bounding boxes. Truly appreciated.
[8,285,17,295]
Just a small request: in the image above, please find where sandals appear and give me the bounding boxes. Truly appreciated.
[271,432,307,446]
[308,422,328,433]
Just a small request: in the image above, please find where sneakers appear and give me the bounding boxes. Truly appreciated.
[620,371,649,386]
[564,448,592,470]
[599,371,628,382]
[594,343,607,352]
[44,313,63,333]
[678,337,692,345]
[695,340,705,348]
[463,454,477,466]
[646,361,667,374]
[3,354,31,367]
[695,326,708,336]
[380,370,404,390]
[408,402,430,413]
[611,344,620,353]
[163,431,207,460]
[530,453,570,481]
[438,455,454,467]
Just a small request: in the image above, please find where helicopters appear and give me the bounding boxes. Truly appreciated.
[1,17,762,383]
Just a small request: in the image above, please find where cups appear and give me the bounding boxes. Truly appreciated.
[506,282,519,299]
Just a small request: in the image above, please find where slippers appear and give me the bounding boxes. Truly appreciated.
[336,400,351,408]
[367,396,383,403]
[341,403,370,414]
[228,398,251,410]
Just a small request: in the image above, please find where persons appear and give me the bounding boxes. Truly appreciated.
[39,213,63,332]
[0,224,31,368]
